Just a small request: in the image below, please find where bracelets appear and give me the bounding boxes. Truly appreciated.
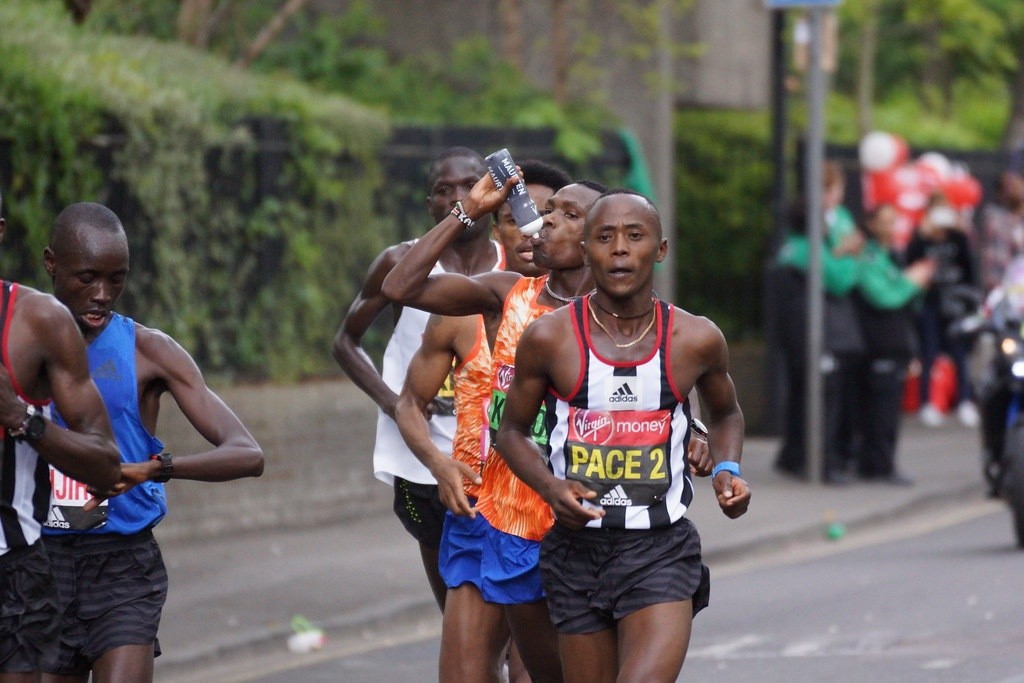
[451,201,474,230]
[150,451,173,483]
[713,462,742,478]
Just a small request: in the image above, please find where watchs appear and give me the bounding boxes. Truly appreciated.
[690,418,708,440]
[9,401,44,443]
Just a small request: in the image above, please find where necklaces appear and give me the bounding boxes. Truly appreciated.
[588,291,656,348]
[545,276,594,302]
[591,288,658,319]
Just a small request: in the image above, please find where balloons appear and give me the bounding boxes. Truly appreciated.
[860,129,982,250]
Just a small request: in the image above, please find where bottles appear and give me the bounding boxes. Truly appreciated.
[288,628,324,654]
[484,148,543,239]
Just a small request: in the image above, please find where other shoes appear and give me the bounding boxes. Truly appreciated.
[856,462,912,486]
[775,446,836,482]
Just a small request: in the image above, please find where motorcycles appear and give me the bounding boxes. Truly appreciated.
[982,286,1024,552]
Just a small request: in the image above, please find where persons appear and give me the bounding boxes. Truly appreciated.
[330,145,506,614]
[44,201,265,683]
[497,187,752,682]
[0,137,122,683]
[397,161,574,683]
[383,164,563,683]
[776,171,1023,498]
